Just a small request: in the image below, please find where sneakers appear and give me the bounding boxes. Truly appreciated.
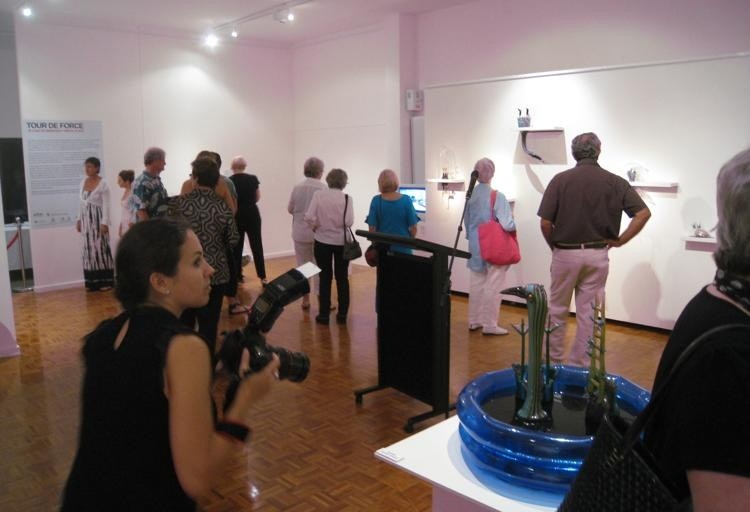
[303,301,310,309]
[262,278,267,287]
[470,322,483,330]
[483,327,508,335]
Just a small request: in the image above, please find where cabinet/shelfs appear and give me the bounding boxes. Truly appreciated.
[423,126,723,246]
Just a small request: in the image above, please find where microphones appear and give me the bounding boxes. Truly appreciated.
[465,170,479,200]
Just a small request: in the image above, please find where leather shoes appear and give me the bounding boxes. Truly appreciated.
[336,312,347,324]
[316,314,329,324]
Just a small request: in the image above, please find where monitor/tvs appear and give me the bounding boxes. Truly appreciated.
[399,187,425,213]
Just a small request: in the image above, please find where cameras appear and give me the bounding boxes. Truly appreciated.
[219,267,310,383]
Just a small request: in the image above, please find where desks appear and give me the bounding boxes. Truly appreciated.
[373,412,564,511]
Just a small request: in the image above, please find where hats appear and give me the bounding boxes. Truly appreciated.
[473,158,496,184]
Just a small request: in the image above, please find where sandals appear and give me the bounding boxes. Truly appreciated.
[229,302,250,313]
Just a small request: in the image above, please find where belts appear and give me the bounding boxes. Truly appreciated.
[554,243,607,249]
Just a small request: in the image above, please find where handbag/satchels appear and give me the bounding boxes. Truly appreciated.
[365,243,378,266]
[558,405,695,511]
[477,222,521,265]
[344,242,362,260]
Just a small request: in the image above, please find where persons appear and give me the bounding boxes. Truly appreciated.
[188,153,240,198]
[463,157,515,335]
[153,156,241,377]
[77,157,115,291]
[116,169,135,237]
[536,132,651,368]
[229,156,270,289]
[653,148,750,511]
[364,168,421,258]
[133,146,168,224]
[180,150,238,219]
[58,219,279,511]
[287,156,337,311]
[303,168,353,324]
